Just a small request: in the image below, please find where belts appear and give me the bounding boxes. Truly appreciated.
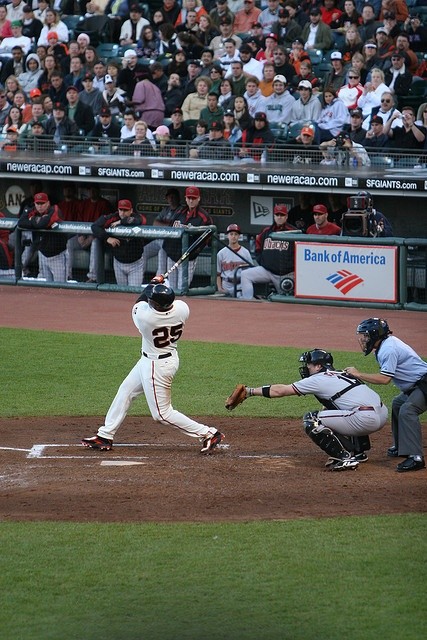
[227,278,240,284]
[358,401,384,410]
[142,352,171,359]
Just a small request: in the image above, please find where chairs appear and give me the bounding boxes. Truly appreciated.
[368,155,393,169]
[93,41,117,58]
[119,43,138,57]
[394,157,420,168]
[305,47,323,64]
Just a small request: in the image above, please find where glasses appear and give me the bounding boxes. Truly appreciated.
[424,110,427,113]
[391,59,400,61]
[1,95,4,98]
[279,16,285,18]
[45,59,54,62]
[101,115,108,118]
[209,70,218,73]
[397,40,406,43]
[275,213,284,216]
[380,99,390,103]
[273,52,283,55]
[225,115,232,116]
[348,76,358,79]
[186,15,196,18]
[241,52,248,54]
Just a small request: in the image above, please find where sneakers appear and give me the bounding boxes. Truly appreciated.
[199,430,225,453]
[325,456,358,471]
[352,451,369,463]
[81,435,113,451]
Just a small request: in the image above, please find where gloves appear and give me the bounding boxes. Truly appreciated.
[163,278,170,287]
[152,274,165,284]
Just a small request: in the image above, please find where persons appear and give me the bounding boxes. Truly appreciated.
[124,67,164,132]
[224,45,264,81]
[217,223,254,299]
[311,89,347,144]
[225,61,251,95]
[209,0,235,31]
[300,6,331,50]
[119,4,150,46]
[261,75,295,136]
[286,62,324,94]
[209,64,222,94]
[162,186,213,290]
[101,74,127,115]
[47,70,68,108]
[321,51,349,93]
[233,0,262,34]
[92,61,106,93]
[357,68,392,120]
[335,133,371,166]
[161,73,185,118]
[359,39,384,82]
[167,107,192,144]
[82,46,99,78]
[64,55,86,92]
[152,125,185,158]
[284,1,310,29]
[257,0,284,34]
[2,106,26,139]
[92,108,120,152]
[0,5,14,39]
[375,11,401,36]
[345,52,372,84]
[257,62,275,96]
[305,204,341,235]
[233,112,274,162]
[78,73,103,119]
[362,92,401,131]
[198,92,224,129]
[243,75,266,118]
[19,100,49,150]
[176,10,199,36]
[173,0,209,29]
[37,9,69,46]
[242,22,265,49]
[181,59,204,95]
[120,110,156,151]
[66,186,115,282]
[217,79,235,114]
[357,191,393,237]
[91,199,147,287]
[272,44,295,81]
[320,0,342,26]
[46,32,69,58]
[199,48,222,76]
[158,23,183,57]
[76,33,90,63]
[17,192,69,282]
[104,60,120,85]
[288,193,319,234]
[241,206,301,299]
[329,0,362,48]
[117,49,153,100]
[34,0,50,25]
[278,126,324,164]
[272,9,302,45]
[53,0,128,20]
[0,212,13,269]
[25,121,57,151]
[151,9,166,33]
[374,27,394,68]
[225,96,253,130]
[384,106,427,158]
[220,38,241,80]
[0,19,32,64]
[113,120,154,157]
[208,14,243,55]
[403,12,427,52]
[255,33,279,65]
[186,121,231,160]
[289,40,311,78]
[22,5,43,45]
[6,0,28,21]
[412,56,427,98]
[30,87,42,104]
[7,180,50,278]
[377,0,408,23]
[245,348,388,471]
[81,274,225,455]
[177,31,204,59]
[142,188,184,276]
[4,75,27,108]
[44,101,77,154]
[64,40,85,74]
[191,121,209,145]
[38,94,54,119]
[164,52,188,77]
[37,55,63,93]
[340,26,362,60]
[344,318,427,472]
[196,15,219,46]
[1,128,23,151]
[0,89,13,127]
[414,103,427,128]
[55,184,83,242]
[62,86,94,144]
[383,50,412,97]
[144,63,168,96]
[4,90,32,125]
[343,108,366,144]
[16,53,44,98]
[319,138,339,165]
[381,34,418,74]
[135,25,160,58]
[2,46,26,86]
[277,80,321,142]
[158,0,181,25]
[36,46,47,69]
[324,193,348,228]
[336,67,364,110]
[181,75,213,120]
[361,116,390,157]
[357,4,384,42]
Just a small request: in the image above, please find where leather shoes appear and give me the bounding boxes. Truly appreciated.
[397,456,425,472]
[388,447,397,457]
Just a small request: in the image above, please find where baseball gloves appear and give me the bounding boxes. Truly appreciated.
[225,384,247,411]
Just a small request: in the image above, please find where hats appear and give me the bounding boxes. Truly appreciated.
[152,124,170,136]
[117,199,132,210]
[375,27,388,36]
[350,109,362,118]
[278,9,289,17]
[265,33,278,41]
[47,31,57,40]
[23,5,32,12]
[254,112,266,121]
[207,121,222,130]
[393,47,404,58]
[369,115,383,123]
[309,7,320,15]
[290,37,304,47]
[363,40,378,48]
[297,80,312,90]
[124,49,137,58]
[271,74,287,84]
[132,71,148,80]
[6,127,16,132]
[31,121,43,127]
[251,22,262,28]
[311,204,327,214]
[273,206,287,215]
[65,86,78,92]
[217,15,230,26]
[77,33,90,45]
[82,72,93,80]
[29,88,41,97]
[301,127,313,136]
[185,187,199,198]
[238,44,251,52]
[329,51,342,60]
[170,107,182,114]
[98,107,110,116]
[128,3,142,12]
[223,109,233,116]
[103,74,113,84]
[172,48,187,58]
[34,193,48,203]
[10,21,22,27]
[52,102,64,110]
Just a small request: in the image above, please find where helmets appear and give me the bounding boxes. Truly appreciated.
[225,223,239,233]
[298,348,335,379]
[143,284,175,308]
[356,317,392,356]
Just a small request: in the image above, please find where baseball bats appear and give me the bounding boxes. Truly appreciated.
[164,228,212,279]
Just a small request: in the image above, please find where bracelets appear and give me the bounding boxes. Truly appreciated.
[262,384,271,398]
